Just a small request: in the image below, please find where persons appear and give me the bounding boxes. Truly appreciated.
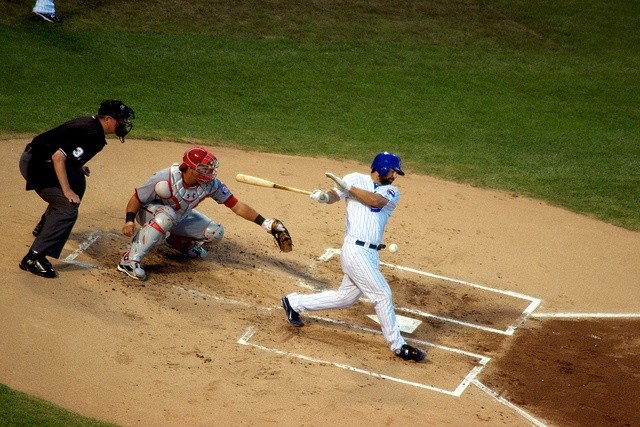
[18,99,135,277]
[281,150,426,362]
[117,147,294,279]
[31,0,60,23]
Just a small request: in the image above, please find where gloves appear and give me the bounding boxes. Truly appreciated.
[325,172,351,193]
[310,189,329,203]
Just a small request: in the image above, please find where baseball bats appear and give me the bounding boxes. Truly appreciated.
[236,174,325,200]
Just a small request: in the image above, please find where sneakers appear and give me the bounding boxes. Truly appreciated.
[34,12,63,24]
[19,250,56,278]
[282,297,303,327]
[166,234,207,259]
[117,251,146,281]
[395,344,426,362]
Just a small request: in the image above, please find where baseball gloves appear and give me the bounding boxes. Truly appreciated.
[270,219,293,252]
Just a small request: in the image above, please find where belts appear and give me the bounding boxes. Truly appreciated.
[355,239,385,250]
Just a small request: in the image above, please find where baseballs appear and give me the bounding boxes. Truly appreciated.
[390,244,398,253]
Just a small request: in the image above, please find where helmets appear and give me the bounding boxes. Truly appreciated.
[99,99,135,143]
[179,148,219,188]
[372,152,404,176]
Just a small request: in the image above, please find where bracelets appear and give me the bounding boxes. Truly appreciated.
[254,214,266,226]
[126,212,135,223]
